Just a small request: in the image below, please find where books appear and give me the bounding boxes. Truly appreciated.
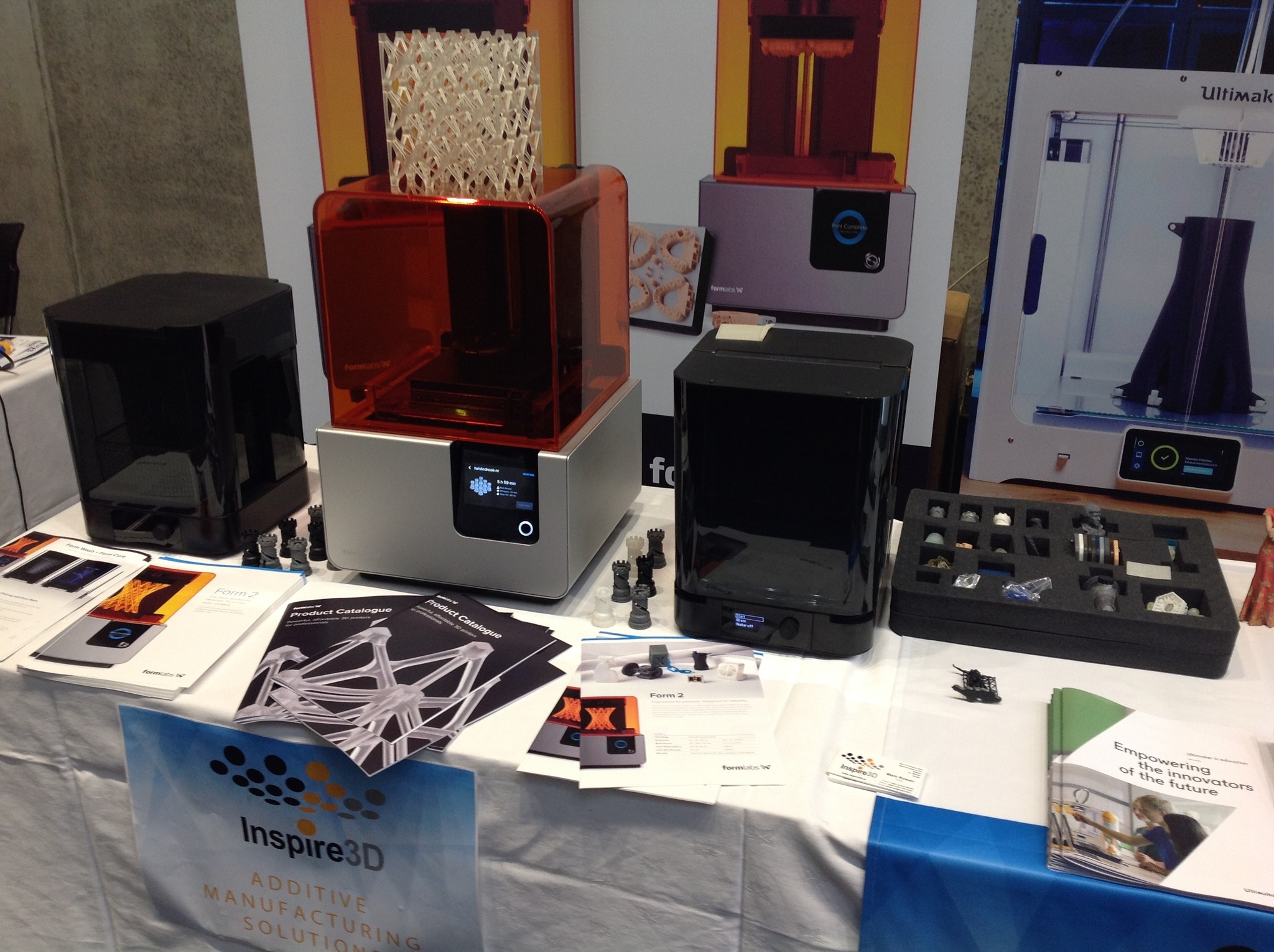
[1047,687,1274,913]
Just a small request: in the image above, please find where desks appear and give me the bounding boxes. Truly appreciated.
[0,338,82,547]
[0,443,1274,952]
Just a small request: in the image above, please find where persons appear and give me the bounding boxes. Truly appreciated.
[1133,797,1163,862]
[1073,795,1208,876]
